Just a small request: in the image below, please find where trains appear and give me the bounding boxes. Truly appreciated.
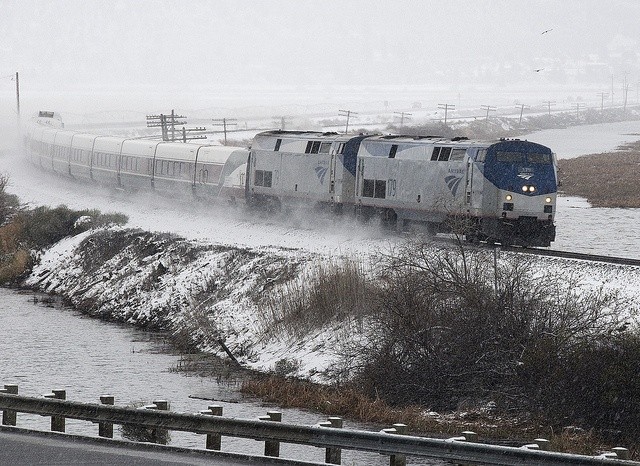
[26,110,556,248]
